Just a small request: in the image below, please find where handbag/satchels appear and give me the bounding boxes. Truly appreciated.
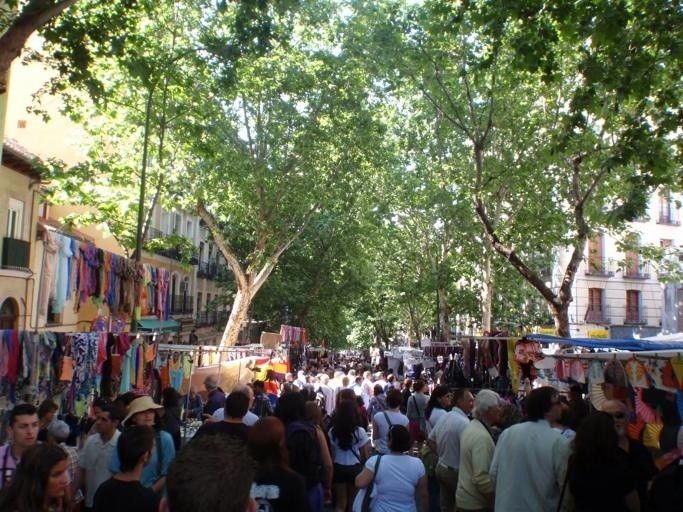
[411,417,425,441]
[352,455,380,512]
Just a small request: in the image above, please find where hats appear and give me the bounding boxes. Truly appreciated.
[121,396,164,427]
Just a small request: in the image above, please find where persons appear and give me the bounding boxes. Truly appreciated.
[4,354,683,512]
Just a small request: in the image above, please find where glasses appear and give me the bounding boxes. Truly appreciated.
[552,400,561,404]
[611,411,626,419]
